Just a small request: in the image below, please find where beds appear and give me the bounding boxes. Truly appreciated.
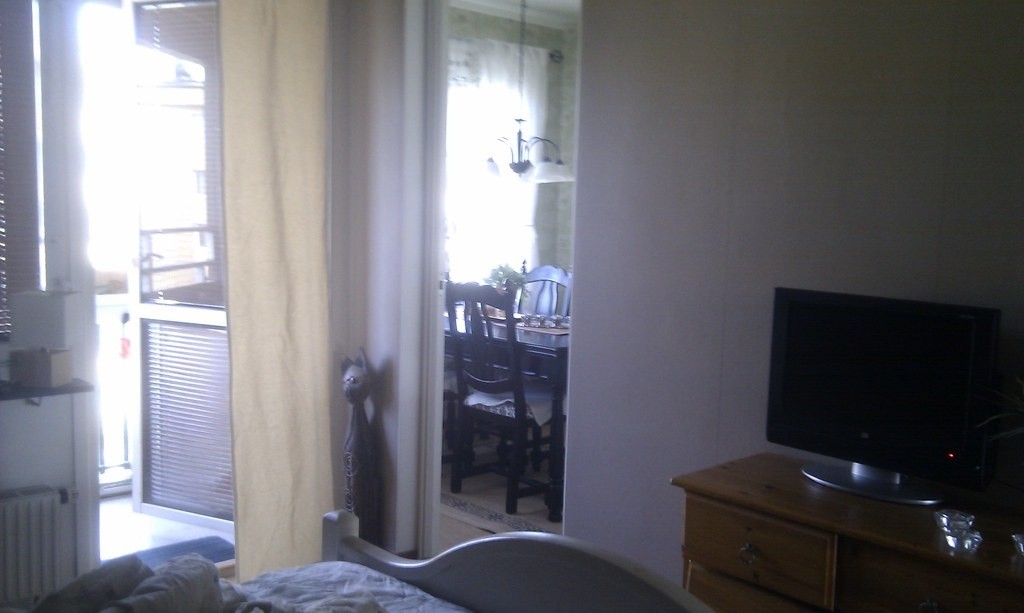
[42,506,717,612]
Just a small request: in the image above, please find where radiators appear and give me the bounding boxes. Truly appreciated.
[0,486,68,607]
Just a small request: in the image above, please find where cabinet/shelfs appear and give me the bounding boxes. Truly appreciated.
[664,451,1024,612]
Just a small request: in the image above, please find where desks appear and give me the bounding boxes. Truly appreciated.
[443,317,570,494]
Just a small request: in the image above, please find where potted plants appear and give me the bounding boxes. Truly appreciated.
[484,264,533,319]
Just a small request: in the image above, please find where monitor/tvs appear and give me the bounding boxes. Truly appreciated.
[767,286,1002,503]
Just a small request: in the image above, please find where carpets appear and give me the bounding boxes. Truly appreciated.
[104,536,237,574]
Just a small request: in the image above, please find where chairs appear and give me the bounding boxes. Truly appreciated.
[440,260,564,520]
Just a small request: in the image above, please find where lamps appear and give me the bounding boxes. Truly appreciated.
[464,1,576,185]
